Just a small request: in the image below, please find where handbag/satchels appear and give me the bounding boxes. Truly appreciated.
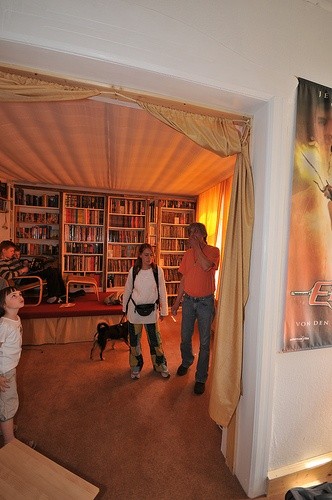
[133,303,154,316]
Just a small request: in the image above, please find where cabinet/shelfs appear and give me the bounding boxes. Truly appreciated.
[11,185,196,305]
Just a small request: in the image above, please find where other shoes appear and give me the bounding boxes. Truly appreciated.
[45,296,71,304]
[161,368,169,378]
[12,424,17,432]
[176,364,187,376]
[193,381,204,395]
[130,370,140,379]
[24,439,37,449]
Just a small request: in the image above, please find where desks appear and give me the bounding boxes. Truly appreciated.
[0,437,100,500]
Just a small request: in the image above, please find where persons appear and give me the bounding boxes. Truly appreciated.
[171,223,220,395]
[0,286,25,445]
[0,241,73,303]
[122,243,170,379]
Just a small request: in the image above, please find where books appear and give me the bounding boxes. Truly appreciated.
[14,186,197,294]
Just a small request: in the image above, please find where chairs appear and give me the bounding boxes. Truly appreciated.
[0,276,45,309]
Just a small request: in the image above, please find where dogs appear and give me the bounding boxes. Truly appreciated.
[90,320,131,361]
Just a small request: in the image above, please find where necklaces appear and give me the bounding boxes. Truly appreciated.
[193,244,206,264]
